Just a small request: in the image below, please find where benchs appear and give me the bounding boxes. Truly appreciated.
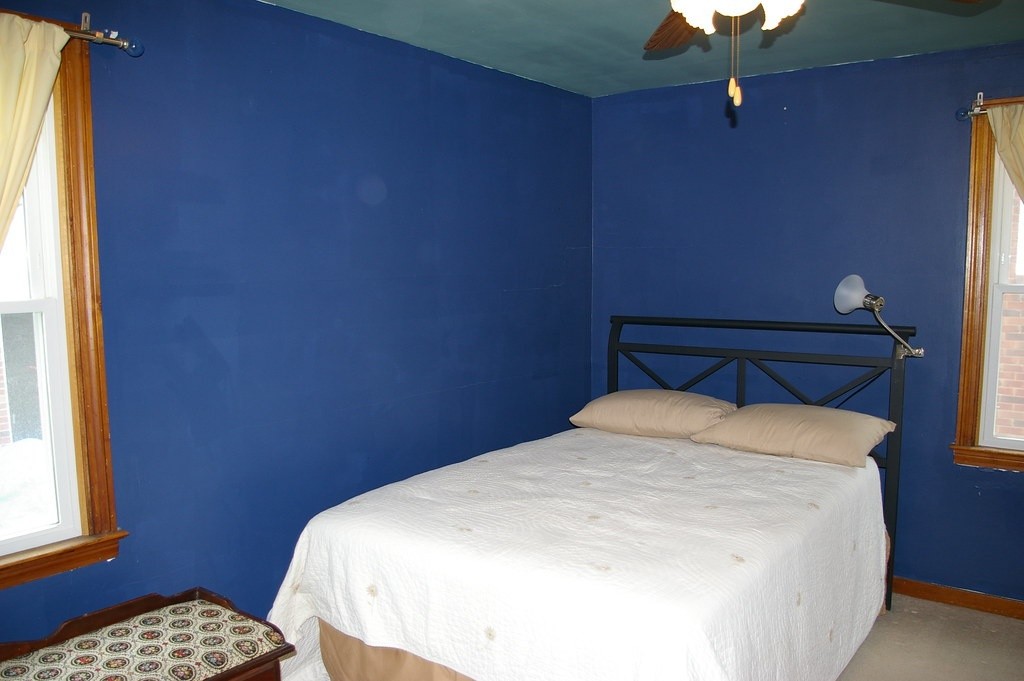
[0,587,297,681]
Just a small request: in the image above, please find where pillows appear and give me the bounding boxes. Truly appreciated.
[690,403,897,468]
[569,389,736,439]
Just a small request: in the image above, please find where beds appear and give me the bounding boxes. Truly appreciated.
[265,315,917,681]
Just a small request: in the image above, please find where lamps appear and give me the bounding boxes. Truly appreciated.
[833,275,925,359]
[643,0,805,106]
